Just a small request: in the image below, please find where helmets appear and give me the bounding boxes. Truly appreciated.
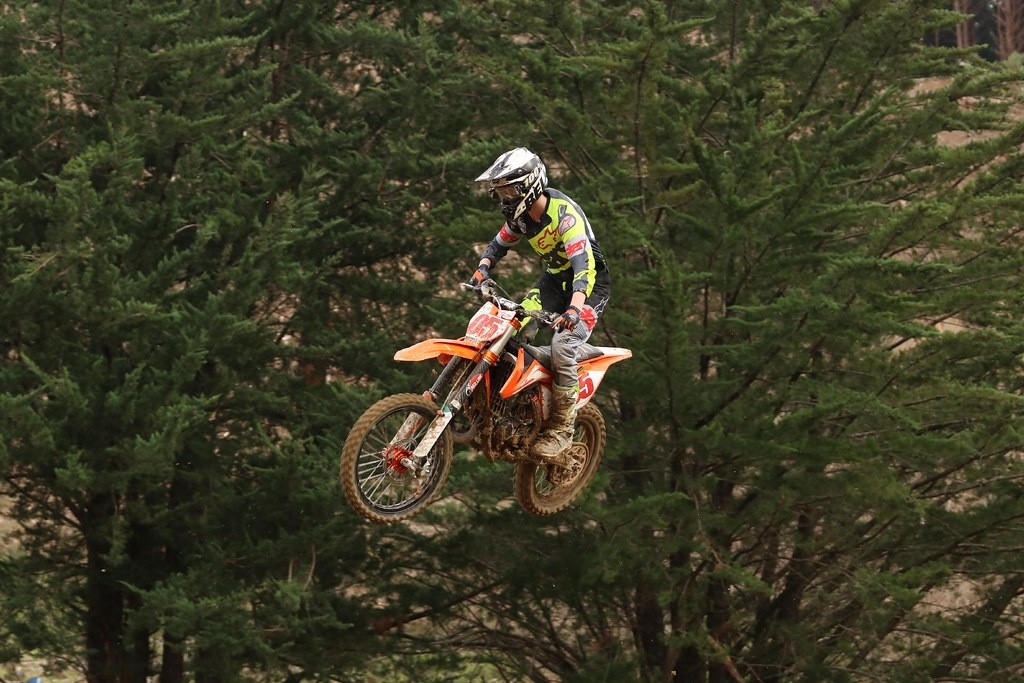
[473,145,549,219]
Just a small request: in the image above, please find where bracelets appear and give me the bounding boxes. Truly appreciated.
[569,305,581,315]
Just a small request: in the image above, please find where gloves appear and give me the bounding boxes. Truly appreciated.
[550,304,582,332]
[469,263,489,286]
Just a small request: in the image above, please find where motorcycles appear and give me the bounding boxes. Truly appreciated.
[339,276,634,525]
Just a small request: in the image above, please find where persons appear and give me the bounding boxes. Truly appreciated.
[463,148,611,456]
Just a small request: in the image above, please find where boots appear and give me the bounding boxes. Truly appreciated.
[531,379,580,458]
